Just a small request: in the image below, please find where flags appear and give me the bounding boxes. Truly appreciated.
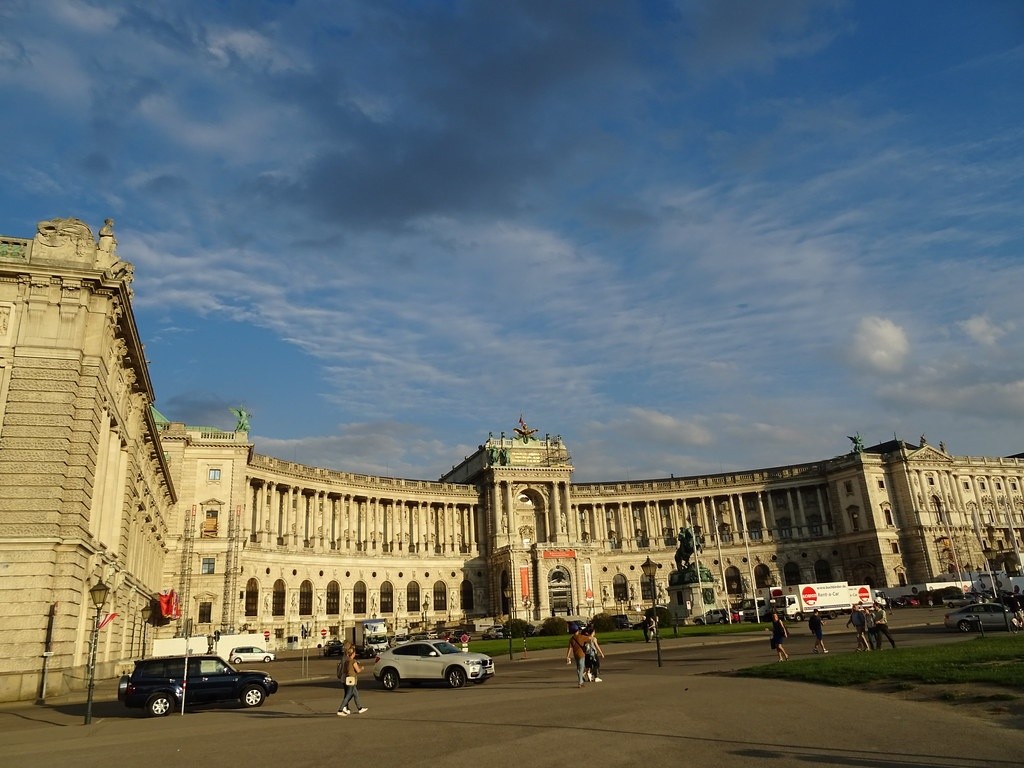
[99,613,117,629]
[519,415,524,426]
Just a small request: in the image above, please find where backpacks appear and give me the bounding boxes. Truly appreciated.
[337,663,343,679]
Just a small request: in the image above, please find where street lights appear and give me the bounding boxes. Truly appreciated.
[503,583,514,660]
[422,601,429,632]
[141,604,153,660]
[641,555,663,668]
[982,547,1012,633]
[83,575,110,721]
[964,562,975,593]
[206,634,213,655]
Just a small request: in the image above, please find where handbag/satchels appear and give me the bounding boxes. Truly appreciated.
[346,676,357,686]
[582,645,587,653]
[595,654,600,668]
[770,638,776,650]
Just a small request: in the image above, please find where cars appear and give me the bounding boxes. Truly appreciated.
[944,603,1024,633]
[373,640,497,688]
[942,594,986,608]
[228,647,276,663]
[323,640,344,656]
[116,653,279,718]
[392,630,468,643]
[692,608,739,627]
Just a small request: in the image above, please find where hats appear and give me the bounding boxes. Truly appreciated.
[571,624,578,630]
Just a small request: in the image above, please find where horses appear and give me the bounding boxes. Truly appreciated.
[675,527,702,570]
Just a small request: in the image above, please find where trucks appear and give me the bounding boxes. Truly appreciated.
[741,581,848,622]
[775,584,873,622]
[870,590,887,610]
[365,630,387,656]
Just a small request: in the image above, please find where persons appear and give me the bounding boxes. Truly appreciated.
[99,218,118,244]
[846,602,896,652]
[1008,592,1024,624]
[642,616,656,644]
[679,527,687,558]
[341,642,355,714]
[236,408,250,432]
[566,625,604,688]
[772,613,789,662]
[810,609,828,654]
[337,648,368,716]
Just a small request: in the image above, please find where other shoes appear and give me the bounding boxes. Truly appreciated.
[343,707,351,715]
[778,655,789,662]
[587,673,591,681]
[578,683,584,688]
[358,707,368,713]
[595,677,602,683]
[337,711,347,716]
[815,648,828,654]
